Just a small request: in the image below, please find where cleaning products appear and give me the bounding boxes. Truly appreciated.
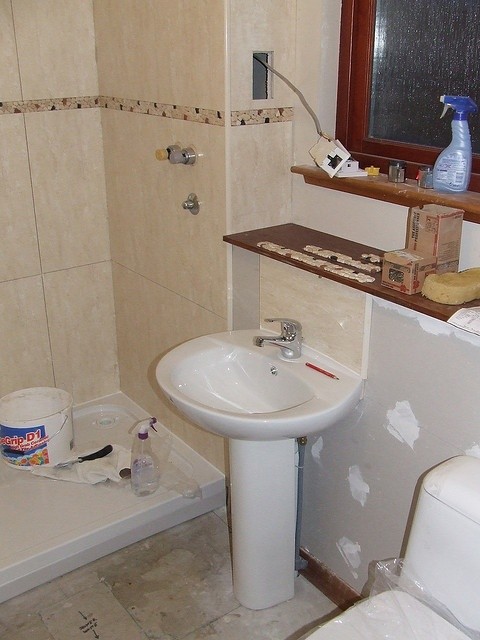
[130,418,160,496]
[432,95,478,194]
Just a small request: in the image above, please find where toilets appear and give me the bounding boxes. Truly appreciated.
[302,457,479,639]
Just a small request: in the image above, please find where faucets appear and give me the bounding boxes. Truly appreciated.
[253,316,306,359]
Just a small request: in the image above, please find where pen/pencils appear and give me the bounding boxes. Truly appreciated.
[306,363,340,380]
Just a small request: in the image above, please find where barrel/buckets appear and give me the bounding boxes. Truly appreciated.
[2,386,74,468]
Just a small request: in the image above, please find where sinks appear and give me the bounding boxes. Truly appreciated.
[170,344,317,418]
